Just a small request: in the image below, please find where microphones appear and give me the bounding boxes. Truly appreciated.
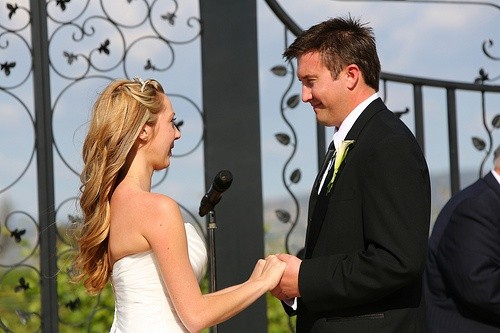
[199,170,233,217]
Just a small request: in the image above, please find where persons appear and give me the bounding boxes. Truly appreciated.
[425,145,500,332]
[67,76,287,333]
[268,11,432,333]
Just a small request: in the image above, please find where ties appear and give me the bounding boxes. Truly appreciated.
[304,137,341,252]
